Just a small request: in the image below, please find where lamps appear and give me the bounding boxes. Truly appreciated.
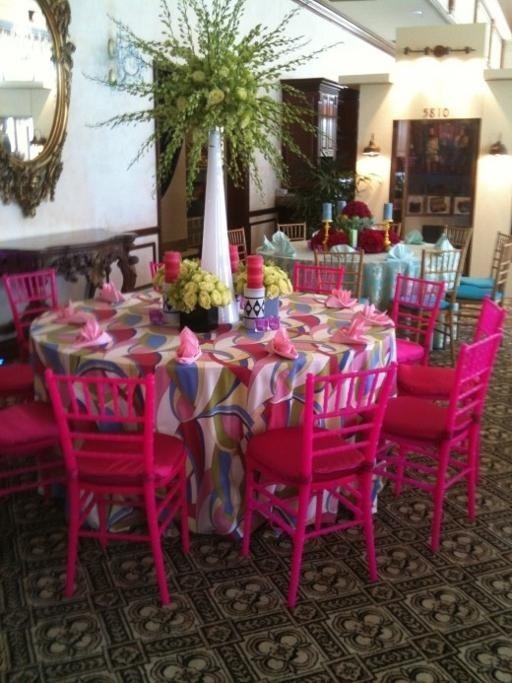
[488,133,508,155]
[360,134,382,158]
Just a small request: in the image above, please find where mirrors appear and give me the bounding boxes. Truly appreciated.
[0,0,76,219]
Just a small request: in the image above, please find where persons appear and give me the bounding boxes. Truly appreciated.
[452,123,470,149]
[424,124,441,173]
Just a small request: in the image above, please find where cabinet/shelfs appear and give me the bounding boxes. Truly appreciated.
[278,77,358,240]
[401,119,480,276]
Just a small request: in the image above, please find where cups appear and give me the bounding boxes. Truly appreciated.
[161,279,182,324]
[243,286,265,329]
[232,270,242,312]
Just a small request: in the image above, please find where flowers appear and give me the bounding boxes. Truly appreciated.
[79,0,332,208]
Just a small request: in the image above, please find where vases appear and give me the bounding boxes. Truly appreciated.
[202,128,240,326]
